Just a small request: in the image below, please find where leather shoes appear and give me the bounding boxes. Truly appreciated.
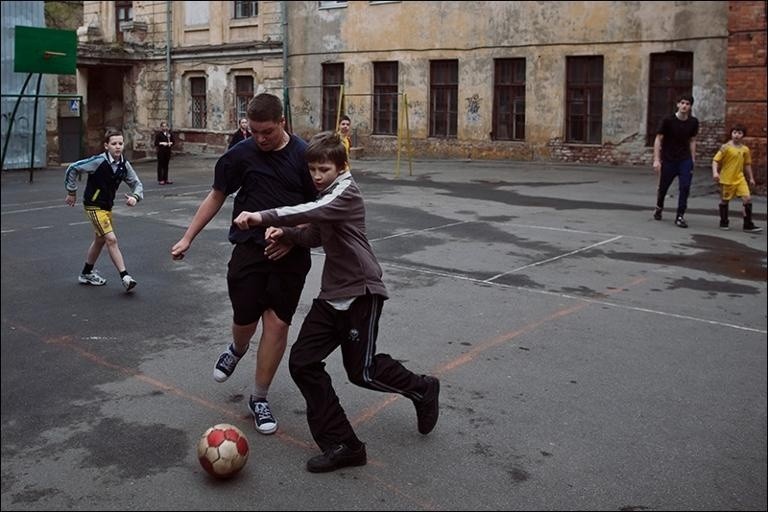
[166,180,173,184]
[159,180,165,185]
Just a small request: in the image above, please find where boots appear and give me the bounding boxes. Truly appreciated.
[743,203,763,232]
[719,202,729,230]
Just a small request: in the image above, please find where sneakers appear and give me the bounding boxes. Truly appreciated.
[653,206,663,221]
[417,374,440,434]
[78,271,106,285]
[674,216,688,228]
[214,343,249,383]
[122,275,137,292]
[308,442,367,472]
[248,394,279,434]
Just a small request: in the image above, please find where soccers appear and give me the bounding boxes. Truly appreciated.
[199,423,250,480]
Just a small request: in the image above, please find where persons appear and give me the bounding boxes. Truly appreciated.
[652,95,699,228]
[172,94,320,434]
[228,117,252,199]
[155,121,173,186]
[713,125,762,233]
[234,132,440,473]
[335,115,351,169]
[65,130,144,292]
